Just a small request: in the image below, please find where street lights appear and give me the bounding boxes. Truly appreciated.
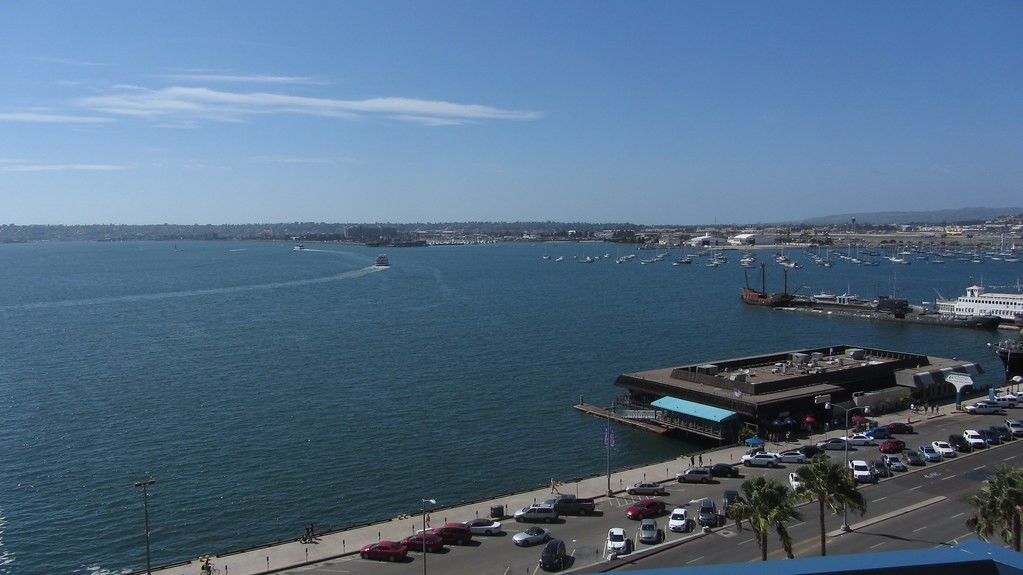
[422,498,436,575]
[824,401,871,531]
[133,478,156,575]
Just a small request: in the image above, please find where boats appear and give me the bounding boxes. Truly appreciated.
[932,276,1023,320]
[298,244,305,250]
[542,232,1023,270]
[374,254,389,267]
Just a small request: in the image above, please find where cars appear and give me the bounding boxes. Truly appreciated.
[398,533,443,553]
[723,489,739,512]
[360,540,408,562]
[794,445,826,459]
[816,437,852,451]
[606,527,628,555]
[460,518,502,536]
[626,499,666,520]
[774,451,806,464]
[839,391,1023,484]
[512,526,552,547]
[637,518,658,544]
[625,480,665,496]
[789,471,806,491]
[668,508,688,532]
[703,463,740,478]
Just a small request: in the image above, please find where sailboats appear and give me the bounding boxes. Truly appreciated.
[739,259,801,308]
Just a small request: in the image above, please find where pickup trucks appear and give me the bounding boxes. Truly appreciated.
[544,494,596,516]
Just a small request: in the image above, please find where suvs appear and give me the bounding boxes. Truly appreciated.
[539,539,567,571]
[676,467,713,484]
[513,504,558,524]
[697,499,719,527]
[740,451,778,468]
[431,523,472,546]
